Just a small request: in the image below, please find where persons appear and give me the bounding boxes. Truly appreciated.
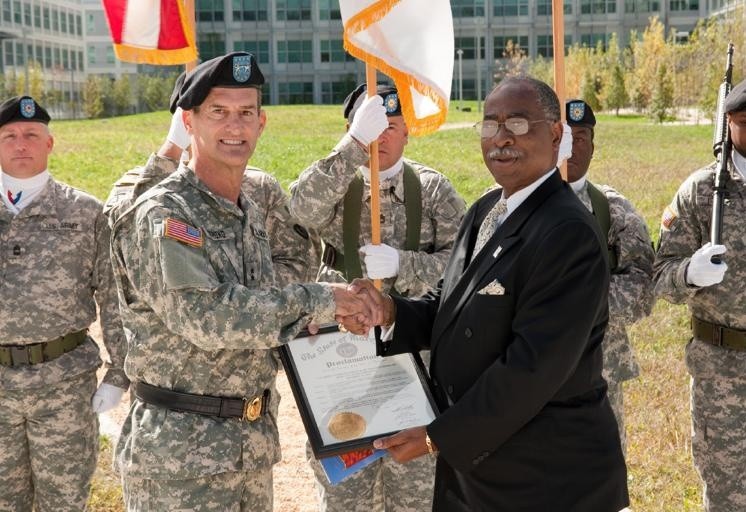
[651,78,746,512]
[288,84,467,512]
[110,52,379,512]
[102,72,313,369]
[556,99,658,464]
[0,94,128,512]
[336,75,630,512]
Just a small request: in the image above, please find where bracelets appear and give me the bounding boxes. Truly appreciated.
[426,432,433,454]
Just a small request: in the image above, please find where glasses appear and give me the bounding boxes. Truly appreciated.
[473,117,545,137]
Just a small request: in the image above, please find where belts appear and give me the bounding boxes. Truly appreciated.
[0,328,87,370]
[135,382,271,423]
[692,316,746,353]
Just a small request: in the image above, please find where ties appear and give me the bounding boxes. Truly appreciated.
[469,200,507,265]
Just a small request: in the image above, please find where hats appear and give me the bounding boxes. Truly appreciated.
[170,52,264,113]
[343,83,402,125]
[566,100,595,128]
[0,96,51,128]
[724,80,746,114]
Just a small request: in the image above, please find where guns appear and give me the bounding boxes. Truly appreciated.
[710,43,734,264]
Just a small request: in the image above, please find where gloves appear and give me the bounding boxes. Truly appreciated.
[359,243,399,280]
[687,242,729,287]
[168,106,191,150]
[555,124,574,169]
[92,384,124,414]
[348,94,389,147]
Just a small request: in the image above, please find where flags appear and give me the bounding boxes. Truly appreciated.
[101,0,198,65]
[336,0,454,138]
